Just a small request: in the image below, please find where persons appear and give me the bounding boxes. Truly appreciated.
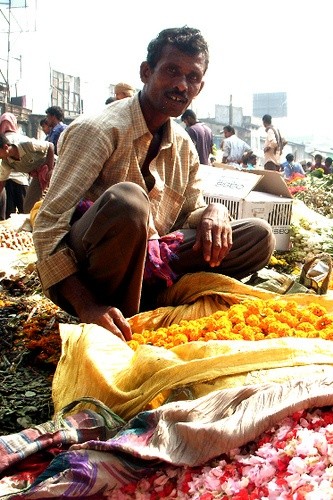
[0,134,59,214]
[221,125,253,170]
[40,118,51,143]
[45,106,68,158]
[283,153,306,178]
[0,111,29,220]
[302,161,313,173]
[246,154,257,170]
[311,154,328,174]
[263,114,287,171]
[105,83,134,105]
[325,157,333,174]
[181,109,213,166]
[32,26,274,343]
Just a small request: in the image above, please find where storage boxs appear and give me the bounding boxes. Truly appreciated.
[194,163,294,251]
